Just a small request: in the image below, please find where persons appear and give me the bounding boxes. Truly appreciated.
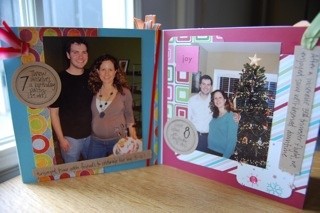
[188,75,241,153]
[87,54,138,160]
[207,90,239,161]
[49,40,93,164]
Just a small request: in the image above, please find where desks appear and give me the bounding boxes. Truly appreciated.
[0,150,320,213]
